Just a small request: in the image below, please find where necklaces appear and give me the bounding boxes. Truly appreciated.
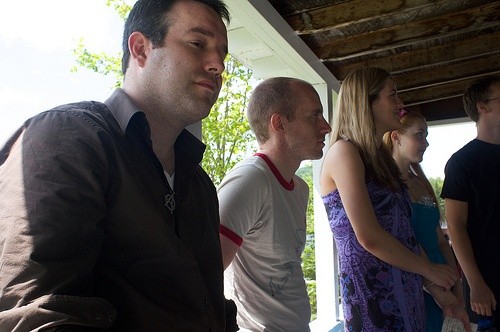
[402,172,415,188]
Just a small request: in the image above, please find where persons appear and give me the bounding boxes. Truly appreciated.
[320,68,464,332]
[217,77,333,332]
[442,78,500,332]
[382,108,467,332]
[0,0,227,332]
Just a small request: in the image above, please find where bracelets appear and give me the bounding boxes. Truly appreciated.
[424,280,434,289]
